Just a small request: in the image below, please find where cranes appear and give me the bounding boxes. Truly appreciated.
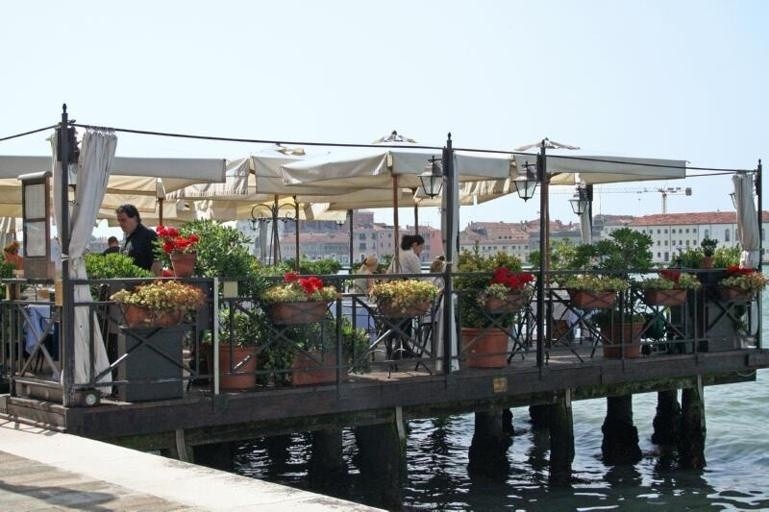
[596,185,691,214]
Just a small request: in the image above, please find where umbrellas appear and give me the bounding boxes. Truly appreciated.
[731,172,760,334]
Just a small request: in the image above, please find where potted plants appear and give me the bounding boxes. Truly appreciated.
[85,218,769,393]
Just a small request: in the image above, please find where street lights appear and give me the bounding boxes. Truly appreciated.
[569,183,593,253]
[416,133,453,377]
[250,202,298,270]
[511,138,548,366]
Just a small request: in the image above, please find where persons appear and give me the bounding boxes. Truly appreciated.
[355,234,444,358]
[2,241,23,267]
[102,203,161,396]
